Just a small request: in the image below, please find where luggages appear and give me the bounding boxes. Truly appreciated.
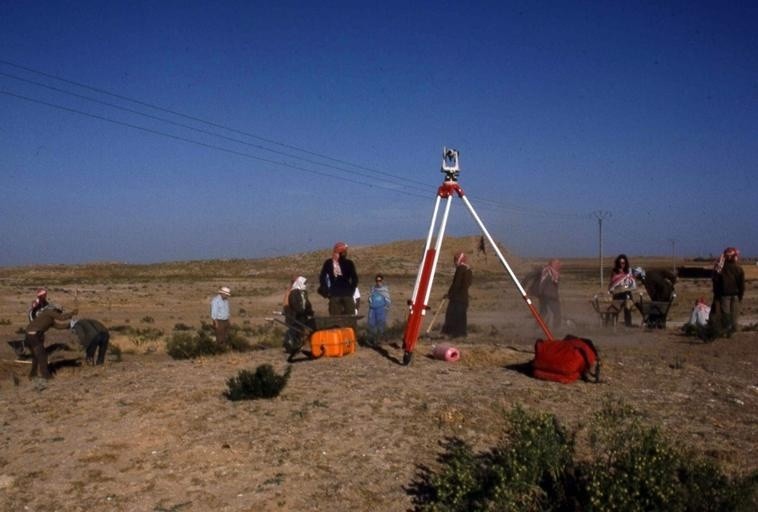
[309,325,357,360]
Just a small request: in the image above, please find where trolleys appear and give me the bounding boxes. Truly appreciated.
[264,311,364,361]
[589,293,676,330]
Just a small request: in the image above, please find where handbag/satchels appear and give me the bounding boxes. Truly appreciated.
[531,334,600,386]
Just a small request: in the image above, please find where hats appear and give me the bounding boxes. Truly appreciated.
[218,286,233,297]
[37,290,46,297]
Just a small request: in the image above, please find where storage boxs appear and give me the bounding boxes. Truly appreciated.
[310,326,356,357]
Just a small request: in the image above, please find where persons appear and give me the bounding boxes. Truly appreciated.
[210,286,231,352]
[288,276,314,350]
[367,275,391,339]
[540,256,562,332]
[318,274,361,315]
[319,241,359,316]
[718,247,745,338]
[609,254,639,328]
[706,252,730,342]
[632,268,681,329]
[685,296,712,337]
[31,289,48,308]
[283,280,300,354]
[439,252,472,341]
[24,306,79,380]
[518,266,547,329]
[70,317,111,367]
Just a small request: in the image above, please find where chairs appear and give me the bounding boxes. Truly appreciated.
[592,293,678,332]
